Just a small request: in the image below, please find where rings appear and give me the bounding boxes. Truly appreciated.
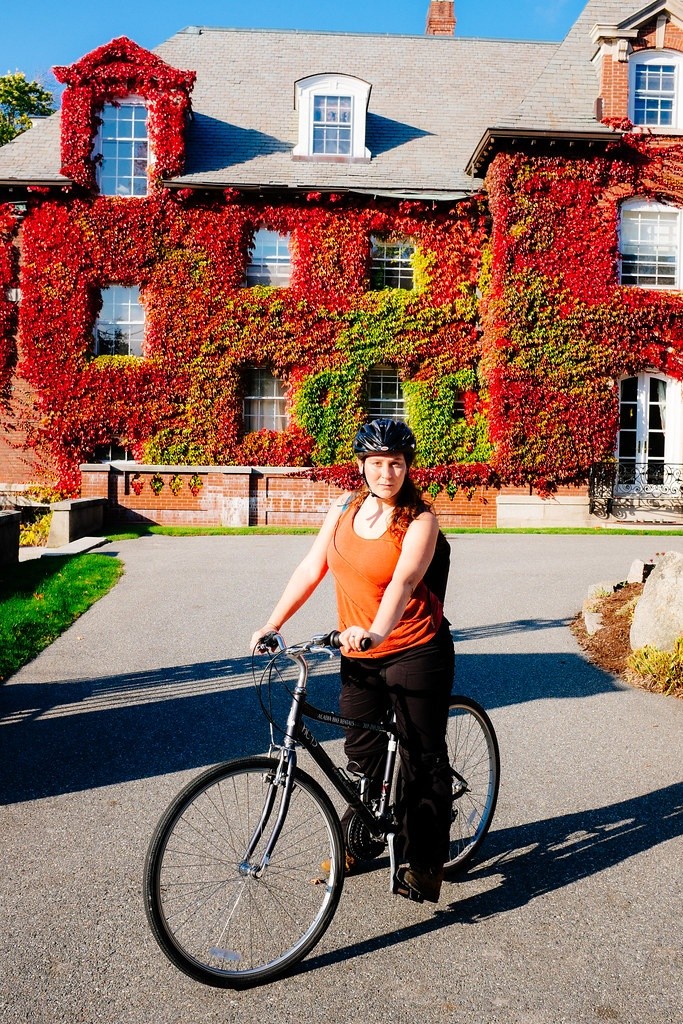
[350,635,355,638]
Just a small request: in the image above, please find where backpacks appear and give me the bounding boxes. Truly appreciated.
[423,529,451,606]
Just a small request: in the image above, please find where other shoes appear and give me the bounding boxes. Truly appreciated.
[322,850,370,875]
[395,863,442,903]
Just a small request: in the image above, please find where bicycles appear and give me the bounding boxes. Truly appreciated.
[141,632,501,990]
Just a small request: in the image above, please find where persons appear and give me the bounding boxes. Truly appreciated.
[250,419,456,903]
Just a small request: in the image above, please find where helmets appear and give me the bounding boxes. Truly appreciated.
[353,418,416,469]
